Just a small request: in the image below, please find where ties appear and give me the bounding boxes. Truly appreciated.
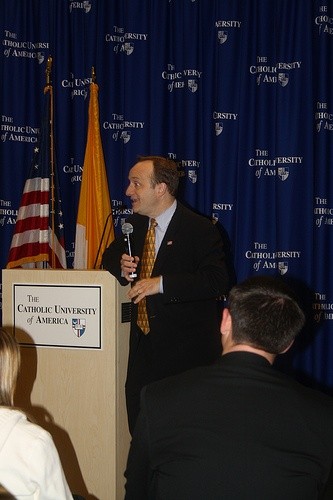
[136,218,158,337]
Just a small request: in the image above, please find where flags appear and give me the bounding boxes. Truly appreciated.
[74,84,116,272]
[6,83,69,270]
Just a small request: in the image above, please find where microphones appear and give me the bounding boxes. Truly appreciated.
[93,204,127,269]
[122,223,137,278]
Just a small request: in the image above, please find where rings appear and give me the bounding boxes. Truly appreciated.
[137,290,140,295]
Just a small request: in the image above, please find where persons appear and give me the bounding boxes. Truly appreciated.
[102,156,237,444]
[0,328,75,499]
[123,279,333,500]
